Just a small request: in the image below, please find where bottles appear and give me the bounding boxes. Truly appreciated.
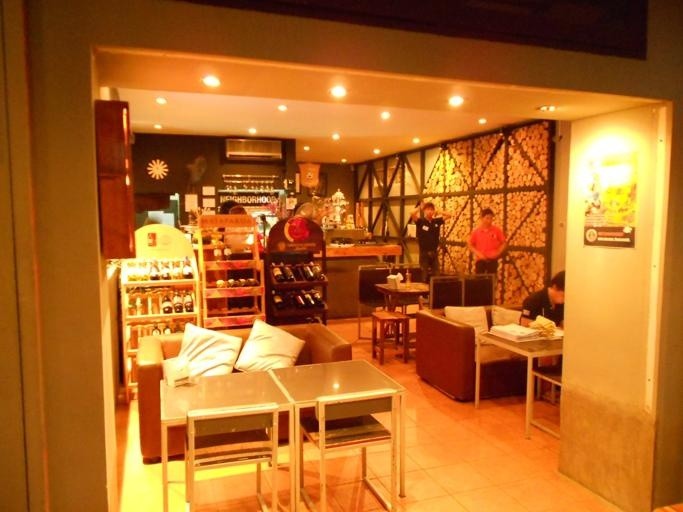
[127,256,193,282]
[271,288,321,308]
[269,261,324,283]
[127,288,193,351]
[129,357,137,382]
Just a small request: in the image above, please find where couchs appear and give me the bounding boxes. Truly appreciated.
[132,318,355,463]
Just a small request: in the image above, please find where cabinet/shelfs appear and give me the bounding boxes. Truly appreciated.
[261,212,334,324]
[93,96,139,260]
[191,209,269,331]
[118,220,207,405]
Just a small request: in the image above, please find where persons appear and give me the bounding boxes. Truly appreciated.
[469,209,506,278]
[217,201,239,242]
[413,203,451,304]
[519,271,565,329]
[221,205,264,253]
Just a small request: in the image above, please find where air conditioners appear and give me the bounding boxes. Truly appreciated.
[222,137,285,164]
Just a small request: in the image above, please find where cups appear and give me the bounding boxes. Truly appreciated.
[404,269,412,288]
[346,224,354,230]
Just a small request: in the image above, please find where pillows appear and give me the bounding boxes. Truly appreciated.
[231,315,301,374]
[174,318,241,379]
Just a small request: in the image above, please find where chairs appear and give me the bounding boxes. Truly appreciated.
[175,395,283,511]
[350,260,562,443]
[297,385,402,511]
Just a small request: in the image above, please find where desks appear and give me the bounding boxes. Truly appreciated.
[268,358,409,512]
[151,367,295,512]
